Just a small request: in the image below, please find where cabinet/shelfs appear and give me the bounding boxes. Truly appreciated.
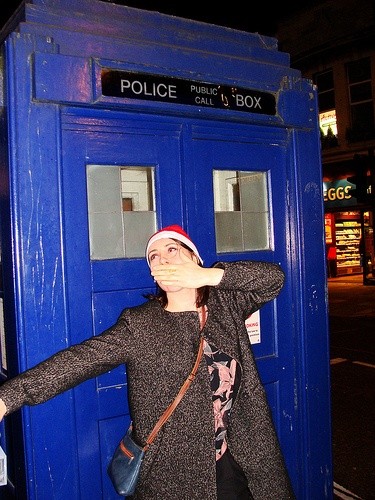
[324,205,372,278]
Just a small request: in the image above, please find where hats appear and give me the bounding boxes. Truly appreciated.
[145,224,203,271]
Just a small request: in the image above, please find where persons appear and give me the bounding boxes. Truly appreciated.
[0,223,297,499]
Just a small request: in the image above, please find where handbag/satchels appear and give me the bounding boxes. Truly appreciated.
[107,420,150,496]
